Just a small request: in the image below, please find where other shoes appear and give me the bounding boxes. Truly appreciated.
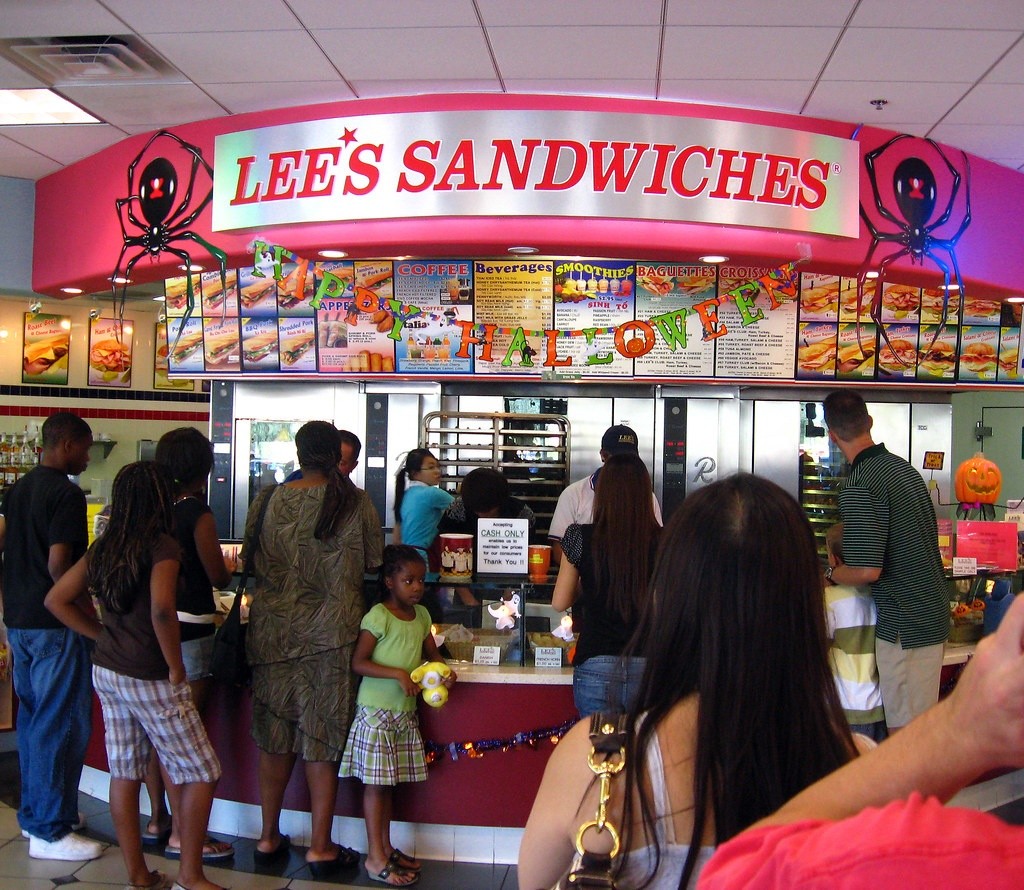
[170,881,230,890]
[124,870,165,890]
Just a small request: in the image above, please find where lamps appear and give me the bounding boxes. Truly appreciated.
[89,296,102,321]
[29,298,42,314]
[158,301,166,323]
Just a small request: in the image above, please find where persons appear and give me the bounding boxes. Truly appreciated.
[822,525,888,743]
[0,412,102,861]
[238,421,386,877]
[285,430,361,484]
[45,461,228,890]
[436,468,536,629]
[547,424,664,567]
[821,390,951,738]
[552,453,662,719]
[144,426,237,857]
[338,545,457,886]
[392,449,455,583]
[514,474,877,890]
[697,587,1024,890]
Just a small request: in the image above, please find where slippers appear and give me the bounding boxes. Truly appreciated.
[308,843,361,870]
[389,848,423,872]
[368,861,418,886]
[143,816,172,839]
[165,835,234,858]
[253,832,291,861]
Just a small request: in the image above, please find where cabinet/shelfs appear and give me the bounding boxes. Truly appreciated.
[420,412,570,573]
[803,461,848,558]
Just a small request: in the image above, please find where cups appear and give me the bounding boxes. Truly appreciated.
[442,533,472,580]
[528,544,552,579]
[219,591,235,612]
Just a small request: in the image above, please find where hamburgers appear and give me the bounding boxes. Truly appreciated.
[360,270,393,292]
[798,282,1023,381]
[90,339,131,373]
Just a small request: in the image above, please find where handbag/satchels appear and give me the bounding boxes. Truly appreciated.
[209,596,252,688]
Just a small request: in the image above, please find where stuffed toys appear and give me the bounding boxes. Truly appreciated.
[412,662,451,707]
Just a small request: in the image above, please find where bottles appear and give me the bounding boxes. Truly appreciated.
[6,452,17,485]
[18,453,27,480]
[20,431,31,465]
[31,432,41,464]
[0,432,11,467]
[11,432,21,464]
[29,453,39,472]
[0,452,5,487]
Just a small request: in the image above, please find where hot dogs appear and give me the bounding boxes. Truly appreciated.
[23,334,69,375]
[164,268,353,365]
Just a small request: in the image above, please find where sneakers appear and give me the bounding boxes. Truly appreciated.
[22,814,86,837]
[29,833,102,861]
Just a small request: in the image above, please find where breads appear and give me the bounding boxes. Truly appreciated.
[531,632,576,648]
[341,350,395,373]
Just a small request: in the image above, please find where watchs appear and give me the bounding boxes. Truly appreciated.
[826,566,836,585]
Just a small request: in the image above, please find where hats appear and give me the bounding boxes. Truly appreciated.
[603,425,638,455]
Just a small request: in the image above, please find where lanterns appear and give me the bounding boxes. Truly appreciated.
[954,458,1002,504]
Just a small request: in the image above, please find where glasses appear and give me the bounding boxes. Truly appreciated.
[420,464,440,471]
